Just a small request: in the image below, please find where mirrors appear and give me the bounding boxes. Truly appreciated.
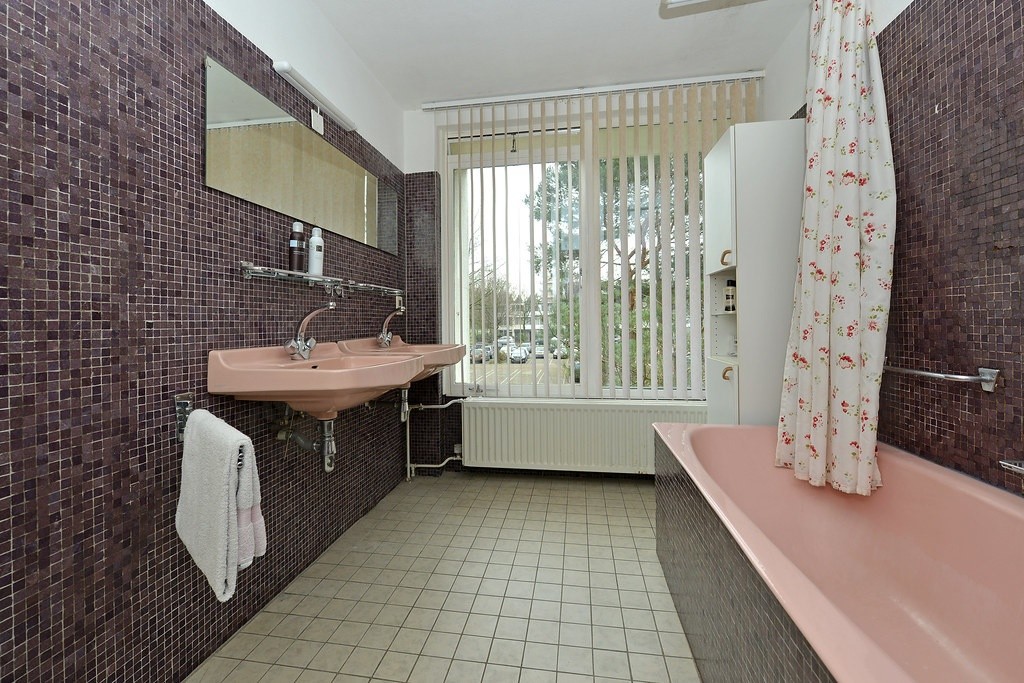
[202,55,399,257]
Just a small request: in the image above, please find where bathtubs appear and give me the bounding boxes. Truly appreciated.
[651,421,1024,683]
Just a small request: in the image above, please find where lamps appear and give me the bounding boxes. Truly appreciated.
[271,60,358,133]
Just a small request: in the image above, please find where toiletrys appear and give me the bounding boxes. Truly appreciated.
[287,221,324,278]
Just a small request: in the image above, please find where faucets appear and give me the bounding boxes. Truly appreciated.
[285,302,337,361]
[376,306,406,347]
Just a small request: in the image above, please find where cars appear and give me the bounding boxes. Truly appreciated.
[470,336,580,382]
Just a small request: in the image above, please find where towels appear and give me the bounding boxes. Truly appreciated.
[174,408,268,602]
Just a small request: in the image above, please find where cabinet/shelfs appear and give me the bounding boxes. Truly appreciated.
[702,116,806,426]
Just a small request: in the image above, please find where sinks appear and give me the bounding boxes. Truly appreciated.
[370,344,466,381]
[265,353,424,412]
